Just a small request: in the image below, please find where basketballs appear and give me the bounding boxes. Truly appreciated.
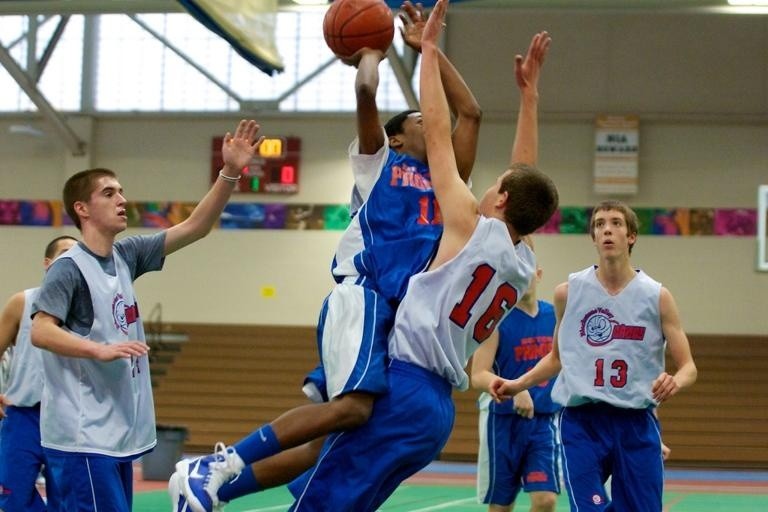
[324,0,394,58]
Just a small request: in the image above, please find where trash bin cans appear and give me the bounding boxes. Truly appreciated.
[141,424,188,482]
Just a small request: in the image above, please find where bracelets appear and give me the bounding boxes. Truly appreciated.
[216,169,244,184]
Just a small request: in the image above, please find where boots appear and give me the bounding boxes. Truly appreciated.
[174,446,244,512]
[168,472,227,512]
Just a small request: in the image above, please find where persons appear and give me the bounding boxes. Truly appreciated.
[468,261,563,512]
[167,0,482,512]
[287,0,554,512]
[29,117,266,512]
[487,198,698,512]
[659,442,672,461]
[0,235,82,512]
